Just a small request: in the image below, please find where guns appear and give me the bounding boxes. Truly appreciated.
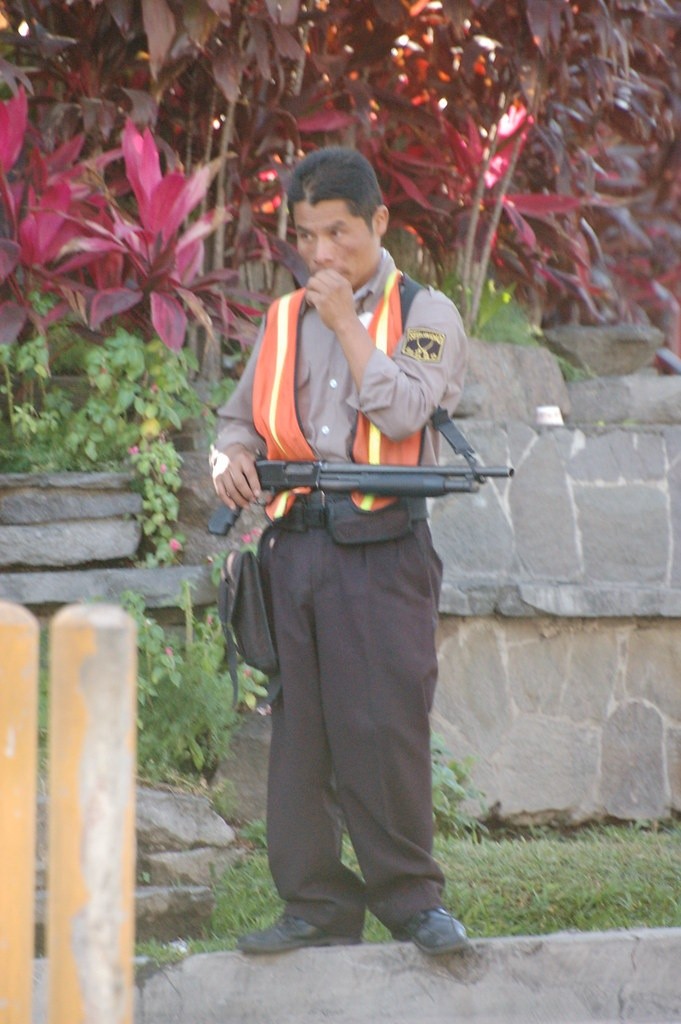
[202,457,517,538]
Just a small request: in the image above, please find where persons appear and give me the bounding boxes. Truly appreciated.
[207,144,467,956]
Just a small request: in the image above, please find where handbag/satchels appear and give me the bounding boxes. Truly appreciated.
[219,549,278,709]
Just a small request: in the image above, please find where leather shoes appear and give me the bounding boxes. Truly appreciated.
[236,915,362,953]
[411,907,466,955]
